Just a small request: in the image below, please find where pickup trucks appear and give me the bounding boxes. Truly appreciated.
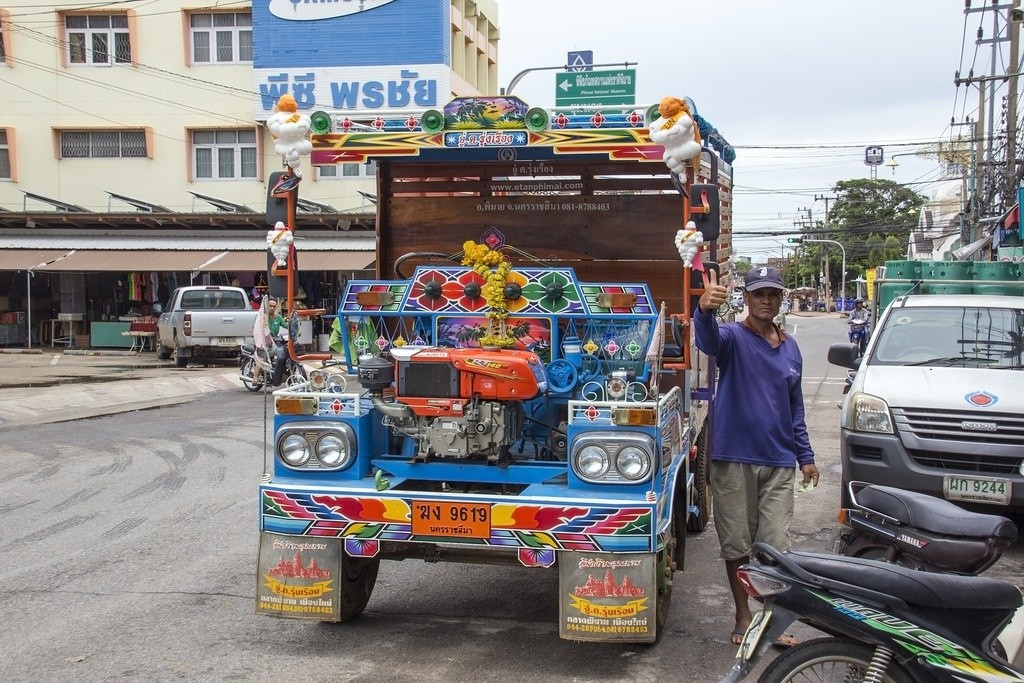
[156,285,260,366]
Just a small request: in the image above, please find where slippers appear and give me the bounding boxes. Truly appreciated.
[774,633,795,647]
[731,629,745,645]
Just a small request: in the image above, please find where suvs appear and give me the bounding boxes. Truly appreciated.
[827,294,1024,542]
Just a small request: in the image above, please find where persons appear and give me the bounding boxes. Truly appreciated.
[252,299,290,384]
[847,297,871,354]
[674,221,703,268]
[693,264,819,648]
[731,295,745,309]
[649,97,701,183]
[266,221,294,267]
[230,275,241,287]
[266,93,313,178]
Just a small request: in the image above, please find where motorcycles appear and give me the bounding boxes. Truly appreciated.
[239,332,308,392]
[824,480,1017,577]
[719,542,1024,683]
[731,301,738,315]
[848,319,871,358]
[737,302,744,314]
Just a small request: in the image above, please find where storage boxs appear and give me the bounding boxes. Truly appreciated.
[57,312,83,321]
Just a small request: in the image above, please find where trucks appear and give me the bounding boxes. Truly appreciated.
[254,92,735,648]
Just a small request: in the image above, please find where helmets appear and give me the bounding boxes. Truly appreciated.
[855,297,864,302]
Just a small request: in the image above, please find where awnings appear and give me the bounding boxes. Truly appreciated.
[0,236,378,349]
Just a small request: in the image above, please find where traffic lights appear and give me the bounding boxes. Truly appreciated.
[788,238,803,243]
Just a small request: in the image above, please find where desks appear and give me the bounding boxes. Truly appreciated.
[50,319,73,349]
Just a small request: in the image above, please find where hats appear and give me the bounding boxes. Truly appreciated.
[745,266,786,291]
